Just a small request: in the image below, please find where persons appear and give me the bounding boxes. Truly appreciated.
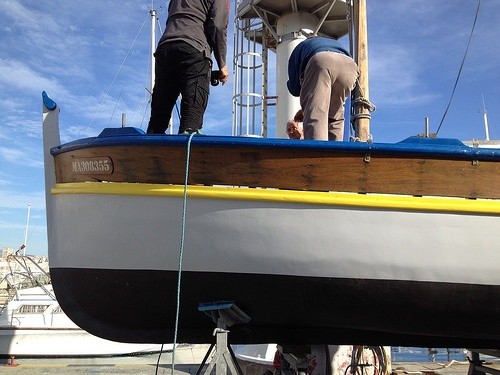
[146,0,231,135]
[286,36,360,141]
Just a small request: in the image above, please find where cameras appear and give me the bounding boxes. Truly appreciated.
[211,71,220,86]
